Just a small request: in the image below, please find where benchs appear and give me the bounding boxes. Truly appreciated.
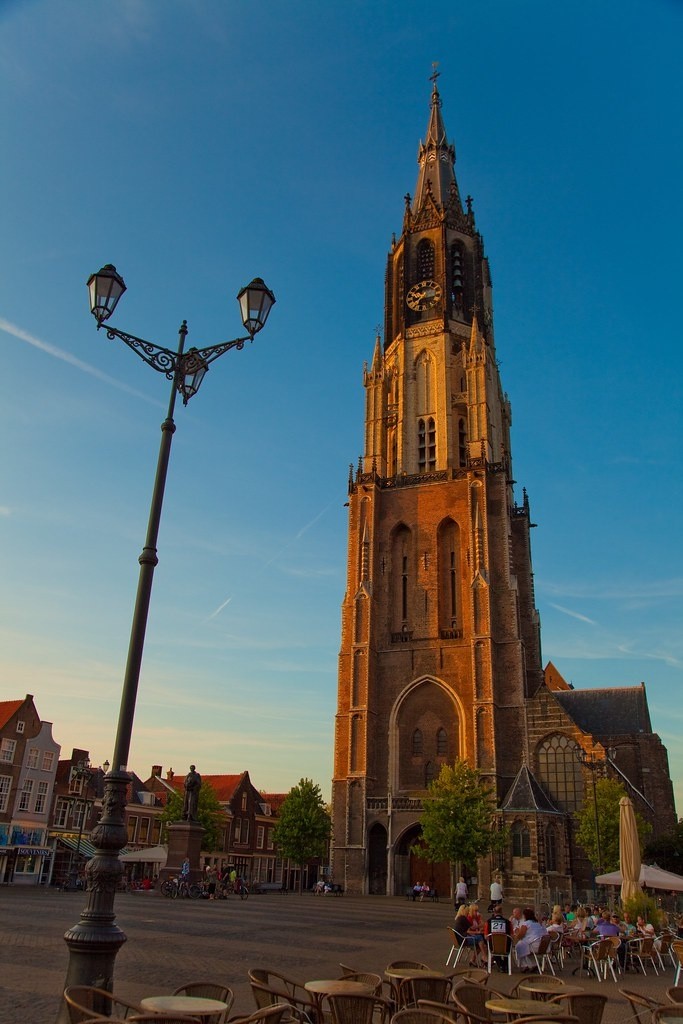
[313,884,344,895]
[257,883,288,894]
[406,887,438,902]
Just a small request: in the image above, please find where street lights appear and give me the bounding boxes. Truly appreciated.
[61,260,276,1024]
[72,757,109,870]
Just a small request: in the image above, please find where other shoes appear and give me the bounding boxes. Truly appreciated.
[522,967,529,974]
[531,966,538,972]
[620,963,629,970]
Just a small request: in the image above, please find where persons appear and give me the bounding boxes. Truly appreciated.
[21,828,37,841]
[467,904,487,945]
[636,915,655,942]
[122,873,151,891]
[420,882,429,902]
[514,908,547,973]
[316,878,339,897]
[70,866,86,886]
[413,881,422,901]
[206,864,256,900]
[657,896,662,904]
[542,903,636,970]
[455,905,488,968]
[678,918,683,936]
[509,907,526,929]
[489,875,505,905]
[182,765,200,821]
[484,906,513,973]
[454,877,468,904]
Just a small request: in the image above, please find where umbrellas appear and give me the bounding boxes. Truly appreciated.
[620,797,646,908]
[120,847,167,881]
[595,865,683,892]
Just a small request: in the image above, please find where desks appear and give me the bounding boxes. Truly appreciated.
[565,935,599,979]
[304,981,375,1024]
[485,999,564,1024]
[384,970,444,991]
[618,937,639,972]
[520,984,583,1004]
[469,931,485,934]
[140,996,228,1024]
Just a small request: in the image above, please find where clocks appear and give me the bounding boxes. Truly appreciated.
[406,281,442,311]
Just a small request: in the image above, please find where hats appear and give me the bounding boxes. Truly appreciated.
[612,912,620,917]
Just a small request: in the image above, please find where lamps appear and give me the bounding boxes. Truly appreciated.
[29,855,32,860]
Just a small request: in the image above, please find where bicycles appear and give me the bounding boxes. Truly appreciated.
[161,874,250,900]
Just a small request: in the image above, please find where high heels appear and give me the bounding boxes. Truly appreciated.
[481,960,488,966]
[469,961,477,967]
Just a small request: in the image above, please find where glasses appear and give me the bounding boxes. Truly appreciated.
[612,916,619,918]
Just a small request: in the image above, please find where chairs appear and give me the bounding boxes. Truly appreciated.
[63,925,683,1024]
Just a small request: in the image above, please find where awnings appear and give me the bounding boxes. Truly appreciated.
[60,837,129,856]
